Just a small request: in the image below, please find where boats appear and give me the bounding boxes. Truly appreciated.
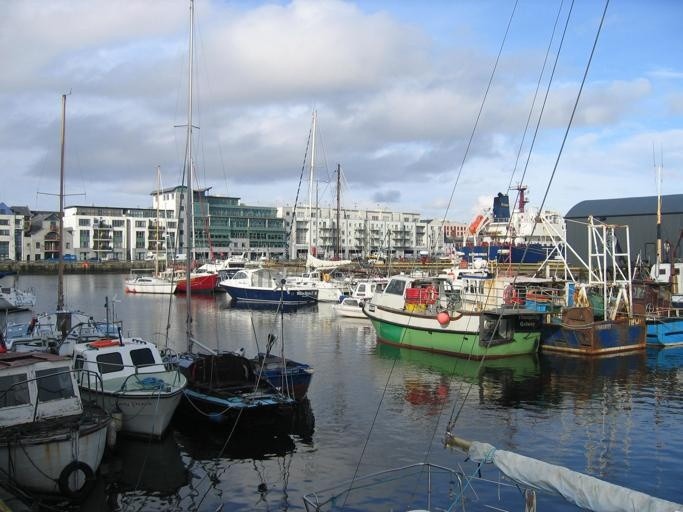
[1,286,38,311]
[247,316,316,402]
[71,296,189,439]
[118,107,682,359]
[0,350,111,500]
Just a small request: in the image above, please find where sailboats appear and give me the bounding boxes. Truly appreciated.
[1,89,125,356]
[161,0,297,437]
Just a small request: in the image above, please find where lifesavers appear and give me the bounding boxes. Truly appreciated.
[503,286,516,303]
[324,274,330,282]
[425,286,438,304]
